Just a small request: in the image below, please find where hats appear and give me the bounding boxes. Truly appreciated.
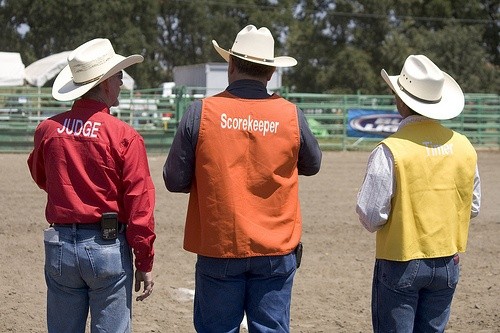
[52,38,145,101]
[212,25,298,67]
[380,54,465,121]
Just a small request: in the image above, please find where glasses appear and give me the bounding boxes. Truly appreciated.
[111,71,124,79]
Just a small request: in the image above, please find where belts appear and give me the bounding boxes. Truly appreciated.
[55,221,126,235]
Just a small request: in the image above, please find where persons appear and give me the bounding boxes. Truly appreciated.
[27,38,156,333]
[356,54,481,333]
[162,25,321,333]
